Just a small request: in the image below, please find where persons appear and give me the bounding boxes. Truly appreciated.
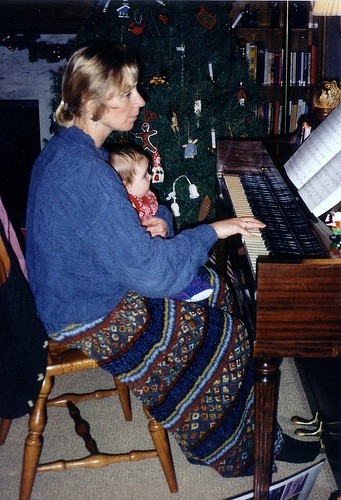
[106,147,159,237]
[23,43,322,479]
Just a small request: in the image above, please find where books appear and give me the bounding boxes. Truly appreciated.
[281,103,341,219]
[243,44,319,144]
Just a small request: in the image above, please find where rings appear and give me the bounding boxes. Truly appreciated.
[243,221,249,229]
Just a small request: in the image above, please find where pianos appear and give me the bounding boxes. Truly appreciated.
[214,135,341,500]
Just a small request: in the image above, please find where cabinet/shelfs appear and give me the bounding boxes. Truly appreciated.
[239,28,319,162]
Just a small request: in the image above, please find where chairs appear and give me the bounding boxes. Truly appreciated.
[0,199,179,500]
